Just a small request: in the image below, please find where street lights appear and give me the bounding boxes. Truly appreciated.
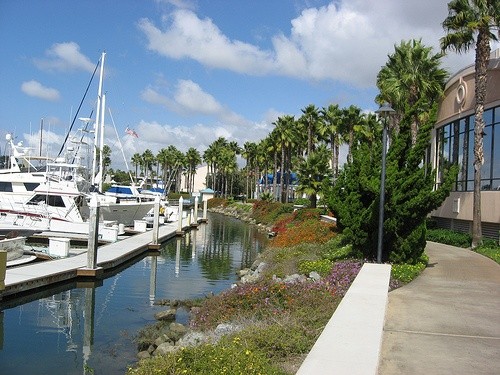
[375,100,398,266]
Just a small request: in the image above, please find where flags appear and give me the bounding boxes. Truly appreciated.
[125,127,133,135]
[132,131,139,139]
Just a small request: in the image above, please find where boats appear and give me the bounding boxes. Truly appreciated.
[0,50,164,259]
